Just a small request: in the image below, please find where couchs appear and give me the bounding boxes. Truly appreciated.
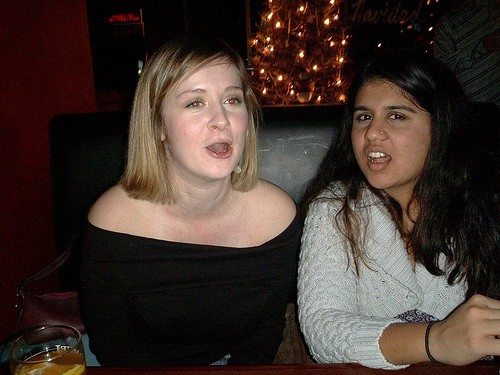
[48,103,491,292]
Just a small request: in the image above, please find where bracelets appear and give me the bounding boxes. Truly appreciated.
[424,320,445,365]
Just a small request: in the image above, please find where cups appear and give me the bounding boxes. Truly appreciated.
[8,324,87,375]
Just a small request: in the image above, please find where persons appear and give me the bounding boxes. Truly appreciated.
[297,49,500,370]
[73,35,299,370]
